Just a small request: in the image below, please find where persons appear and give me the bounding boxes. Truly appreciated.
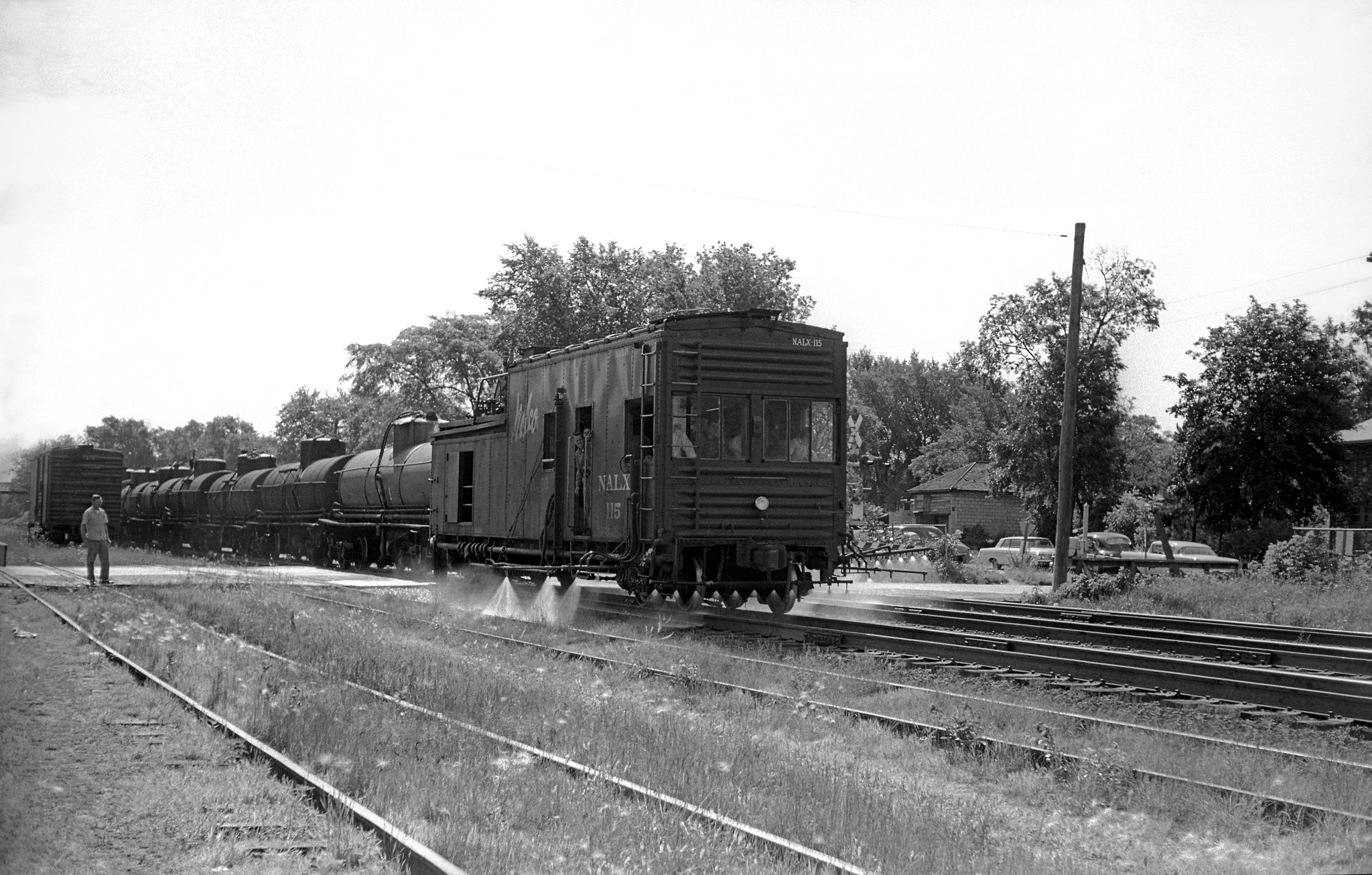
[672,424,698,459]
[784,427,821,462]
[582,428,594,443]
[690,418,724,459]
[80,493,114,586]
[574,431,592,536]
[726,418,761,460]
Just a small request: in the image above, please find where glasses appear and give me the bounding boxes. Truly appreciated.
[93,500,103,503]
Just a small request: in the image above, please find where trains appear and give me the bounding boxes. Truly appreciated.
[29,309,852,613]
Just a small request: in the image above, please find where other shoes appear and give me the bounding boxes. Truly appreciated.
[98,580,115,584]
[90,581,95,585]
